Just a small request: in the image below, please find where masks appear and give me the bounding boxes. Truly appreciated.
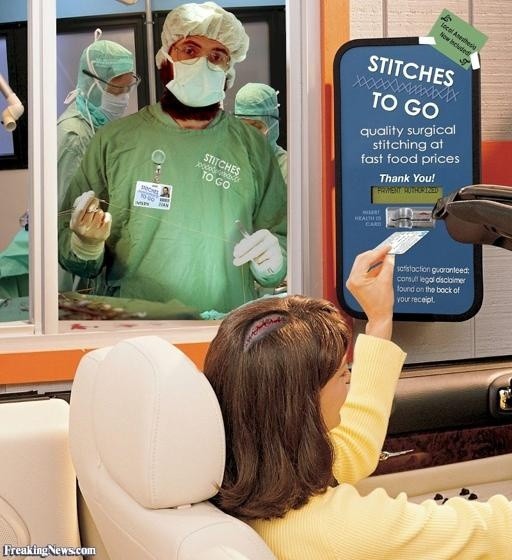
[166,53,232,108]
[98,82,129,116]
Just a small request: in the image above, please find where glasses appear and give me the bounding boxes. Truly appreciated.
[251,122,262,129]
[82,69,142,95]
[173,43,229,72]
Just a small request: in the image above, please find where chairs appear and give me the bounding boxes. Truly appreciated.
[70,336,278,560]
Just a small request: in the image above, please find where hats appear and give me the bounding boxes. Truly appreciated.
[78,40,134,106]
[156,2,249,70]
[235,83,280,122]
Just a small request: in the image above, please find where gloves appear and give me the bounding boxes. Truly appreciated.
[233,229,282,275]
[69,191,111,261]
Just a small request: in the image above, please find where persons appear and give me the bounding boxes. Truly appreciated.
[160,186,170,198]
[57,0,289,314]
[24,38,142,291]
[203,240,512,560]
[233,73,288,197]
